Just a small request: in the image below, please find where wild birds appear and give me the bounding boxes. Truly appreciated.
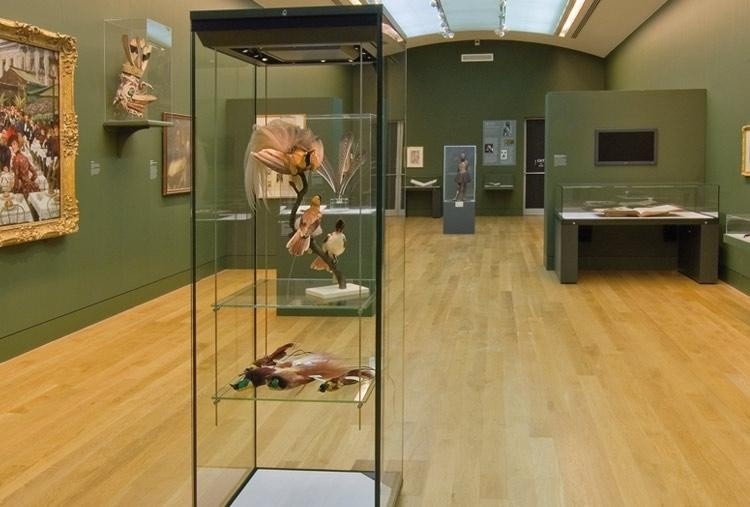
[309,219,347,272]
[243,118,336,216]
[285,193,323,257]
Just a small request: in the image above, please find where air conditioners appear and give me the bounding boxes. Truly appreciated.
[461,53,494,62]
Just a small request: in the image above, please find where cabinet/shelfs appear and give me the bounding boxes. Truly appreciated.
[189,4,407,507]
[720,215,750,297]
[554,185,720,283]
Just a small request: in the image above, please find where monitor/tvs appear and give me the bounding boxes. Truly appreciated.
[594,128,657,166]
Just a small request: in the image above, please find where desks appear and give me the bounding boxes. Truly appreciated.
[405,186,442,218]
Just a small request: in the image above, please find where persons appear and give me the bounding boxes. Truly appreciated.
[0,101,60,201]
[453,149,471,200]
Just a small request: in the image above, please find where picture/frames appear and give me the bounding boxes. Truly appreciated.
[401,146,424,169]
[741,125,750,178]
[161,113,195,197]
[0,18,80,250]
[254,114,307,200]
